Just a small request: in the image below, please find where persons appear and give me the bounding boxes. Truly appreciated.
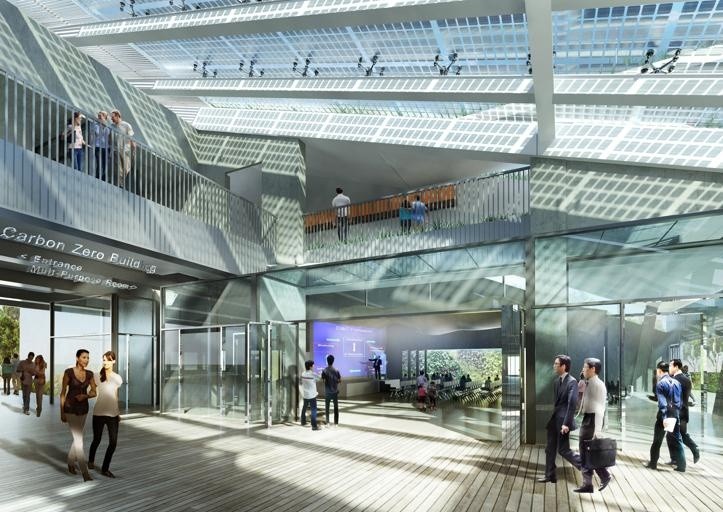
[683,365,698,404]
[299,360,322,430]
[61,111,92,170]
[16,352,35,415]
[60,350,97,482]
[34,355,47,417]
[90,111,110,181]
[537,355,594,483]
[406,195,430,233]
[11,353,21,395]
[402,370,500,412]
[321,355,341,427]
[332,187,351,244]
[572,357,612,493]
[107,109,136,189]
[399,199,413,234]
[1,358,13,395]
[642,361,686,473]
[606,379,621,406]
[660,359,700,464]
[368,356,382,380]
[89,351,122,477]
